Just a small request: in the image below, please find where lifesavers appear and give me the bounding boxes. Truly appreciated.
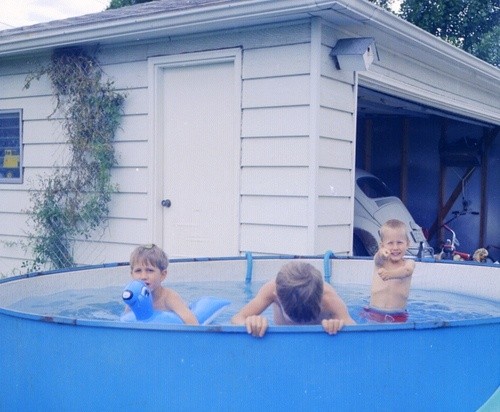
[114,285,231,328]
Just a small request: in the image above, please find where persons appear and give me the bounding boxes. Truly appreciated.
[227,260,359,340]
[122,242,199,326]
[360,216,416,326]
